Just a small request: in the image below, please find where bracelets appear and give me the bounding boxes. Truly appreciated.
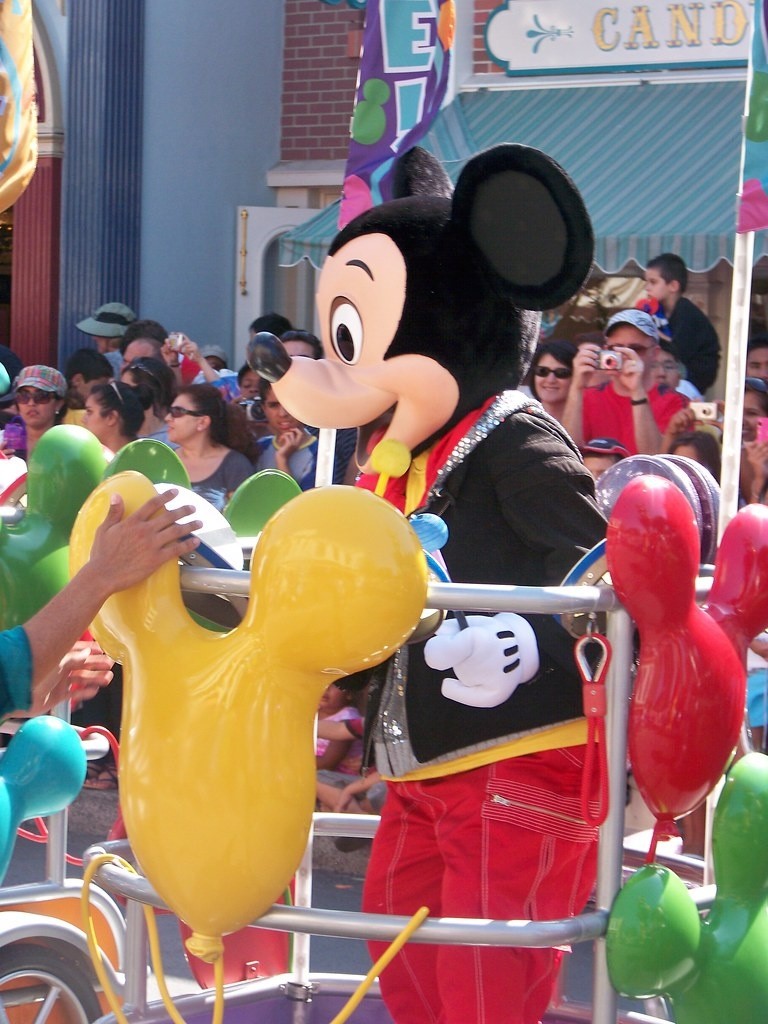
[751,492,760,500]
[631,398,648,406]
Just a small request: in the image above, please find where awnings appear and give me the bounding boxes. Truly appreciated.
[277,72,768,274]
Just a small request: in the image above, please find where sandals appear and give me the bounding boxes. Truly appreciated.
[84,762,118,791]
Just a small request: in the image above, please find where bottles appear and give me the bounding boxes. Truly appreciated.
[4,416,27,461]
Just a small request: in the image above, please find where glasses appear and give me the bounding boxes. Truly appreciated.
[131,356,165,390]
[281,330,318,341]
[15,391,51,405]
[744,377,768,394]
[605,342,656,355]
[169,406,204,418]
[533,366,572,379]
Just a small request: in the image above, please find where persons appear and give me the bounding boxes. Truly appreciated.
[0,303,388,852]
[519,253,768,805]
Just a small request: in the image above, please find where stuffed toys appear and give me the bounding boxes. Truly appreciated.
[246,144,611,1024]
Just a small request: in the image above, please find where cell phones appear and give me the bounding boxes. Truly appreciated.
[4,423,27,461]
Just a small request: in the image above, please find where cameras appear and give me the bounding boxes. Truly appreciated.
[689,401,717,421]
[237,396,268,422]
[169,335,184,350]
[593,350,622,370]
[755,417,768,447]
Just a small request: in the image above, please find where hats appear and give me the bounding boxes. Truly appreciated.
[12,365,68,396]
[603,309,658,340]
[75,303,139,338]
[578,437,631,459]
